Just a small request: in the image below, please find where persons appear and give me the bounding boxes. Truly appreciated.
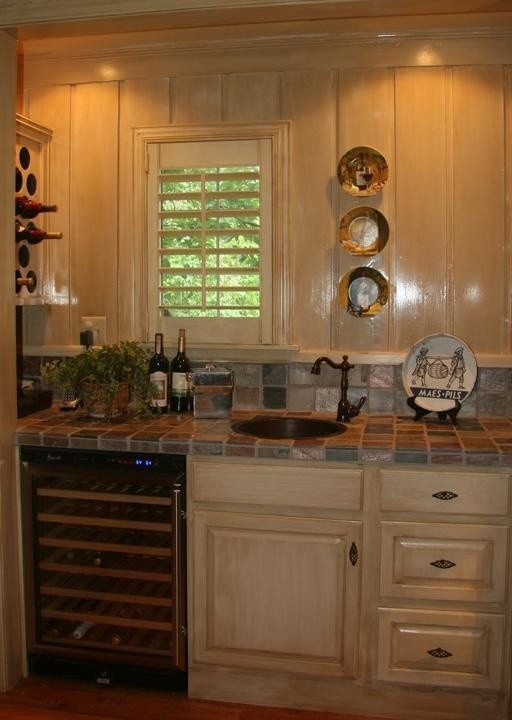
[443,347,467,391]
[410,346,430,386]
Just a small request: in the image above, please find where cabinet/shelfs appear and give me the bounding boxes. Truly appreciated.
[369,459,510,718]
[182,449,370,715]
[14,112,54,308]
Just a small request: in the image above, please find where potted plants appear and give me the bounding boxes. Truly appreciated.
[35,336,165,429]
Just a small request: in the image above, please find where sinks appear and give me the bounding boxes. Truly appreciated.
[231,416,346,440]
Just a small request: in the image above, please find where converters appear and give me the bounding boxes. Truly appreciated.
[79,331,93,346]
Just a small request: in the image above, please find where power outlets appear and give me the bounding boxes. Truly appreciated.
[80,316,107,347]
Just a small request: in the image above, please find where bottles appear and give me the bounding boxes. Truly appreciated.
[13,274,36,289]
[169,328,194,414]
[14,193,66,242]
[147,332,171,415]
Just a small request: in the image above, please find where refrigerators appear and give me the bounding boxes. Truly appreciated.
[16,443,187,696]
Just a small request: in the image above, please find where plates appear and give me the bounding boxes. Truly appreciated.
[337,265,391,321]
[402,334,478,412]
[338,206,392,257]
[335,146,392,198]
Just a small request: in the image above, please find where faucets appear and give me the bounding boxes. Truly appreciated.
[310,354,366,423]
